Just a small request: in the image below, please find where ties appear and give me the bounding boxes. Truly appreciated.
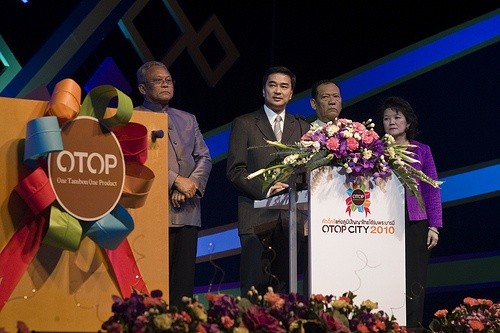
[273,116,282,142]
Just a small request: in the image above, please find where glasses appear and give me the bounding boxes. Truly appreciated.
[142,78,173,86]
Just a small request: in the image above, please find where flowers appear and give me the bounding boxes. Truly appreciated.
[97,283,500,333]
[246,111,445,216]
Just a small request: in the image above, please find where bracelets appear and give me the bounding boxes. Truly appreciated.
[428,227,439,234]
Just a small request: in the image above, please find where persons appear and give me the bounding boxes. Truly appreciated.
[380,98,442,328]
[310,80,342,126]
[134,62,213,309]
[226,67,310,298]
